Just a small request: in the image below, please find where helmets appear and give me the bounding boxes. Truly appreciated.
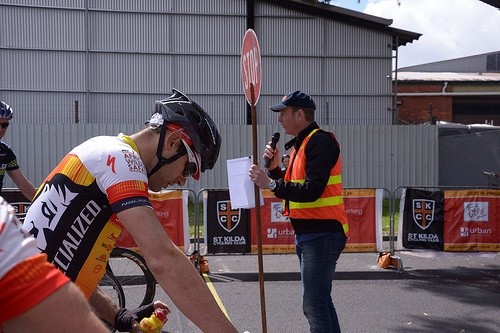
[0,101,13,119]
[155,88,222,181]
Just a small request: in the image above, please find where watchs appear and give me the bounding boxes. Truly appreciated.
[269,180,276,190]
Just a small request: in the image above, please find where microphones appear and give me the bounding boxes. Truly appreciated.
[265,131,281,167]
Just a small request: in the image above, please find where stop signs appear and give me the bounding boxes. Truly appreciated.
[241,29,261,106]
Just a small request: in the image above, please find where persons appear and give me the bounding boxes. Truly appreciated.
[0,197,110,333]
[282,154,290,168]
[0,100,38,207]
[21,86,248,333]
[249,90,349,333]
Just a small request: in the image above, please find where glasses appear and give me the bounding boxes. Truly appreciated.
[181,138,198,179]
[0,122,9,129]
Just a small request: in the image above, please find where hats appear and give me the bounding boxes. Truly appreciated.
[270,91,317,112]
